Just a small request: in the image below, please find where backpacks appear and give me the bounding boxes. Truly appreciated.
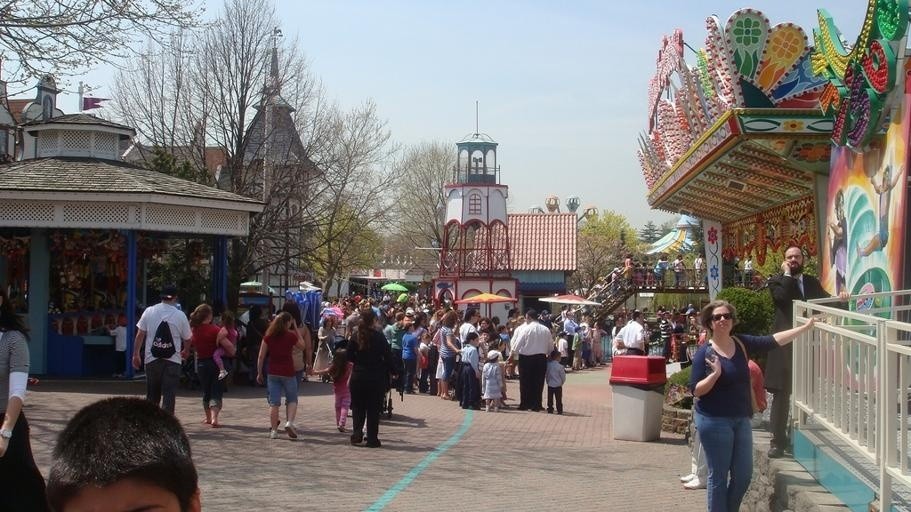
[151,307,176,359]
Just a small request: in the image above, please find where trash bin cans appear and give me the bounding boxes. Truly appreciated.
[609,355,666,441]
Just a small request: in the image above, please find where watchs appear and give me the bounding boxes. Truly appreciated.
[1,428,13,438]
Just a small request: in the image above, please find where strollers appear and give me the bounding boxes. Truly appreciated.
[318,334,347,383]
[348,372,396,419]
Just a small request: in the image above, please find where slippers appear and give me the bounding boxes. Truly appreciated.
[212,424,217,428]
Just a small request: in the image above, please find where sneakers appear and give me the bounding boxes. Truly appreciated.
[217,368,229,381]
[366,439,381,447]
[337,425,346,432]
[680,473,696,483]
[767,443,784,458]
[350,435,363,445]
[283,421,299,438]
[683,479,707,490]
[404,387,563,415]
[270,429,281,439]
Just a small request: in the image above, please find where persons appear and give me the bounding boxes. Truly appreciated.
[763,245,851,458]
[744,255,753,288]
[45,397,201,512]
[764,273,772,288]
[753,270,761,290]
[680,430,709,490]
[734,258,742,287]
[0,278,46,512]
[690,299,820,511]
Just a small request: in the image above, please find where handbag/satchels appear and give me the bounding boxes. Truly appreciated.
[731,335,768,414]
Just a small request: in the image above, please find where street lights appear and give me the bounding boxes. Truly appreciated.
[412,245,442,268]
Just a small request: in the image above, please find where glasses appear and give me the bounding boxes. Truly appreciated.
[710,313,732,321]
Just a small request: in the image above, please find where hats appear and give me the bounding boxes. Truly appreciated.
[487,350,502,360]
[405,309,415,316]
[159,286,177,299]
